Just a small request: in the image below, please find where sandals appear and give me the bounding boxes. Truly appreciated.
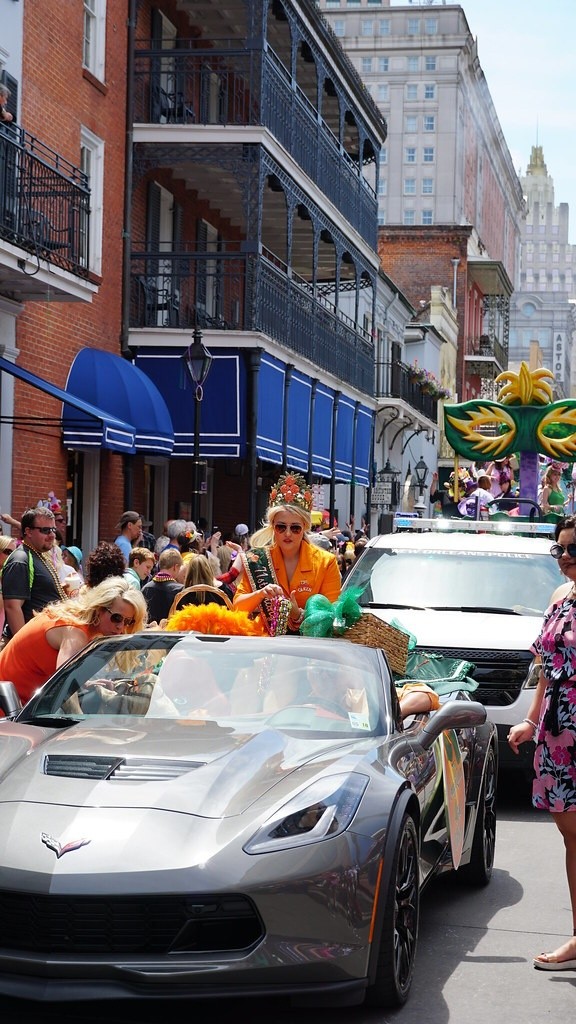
[533,937,576,971]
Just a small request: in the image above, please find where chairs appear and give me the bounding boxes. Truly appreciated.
[152,81,197,126]
[133,270,181,327]
[13,206,82,254]
[192,306,233,329]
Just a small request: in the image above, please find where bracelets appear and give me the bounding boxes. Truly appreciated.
[292,608,305,623]
[523,719,537,730]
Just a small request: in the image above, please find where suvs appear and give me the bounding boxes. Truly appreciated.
[329,499,573,796]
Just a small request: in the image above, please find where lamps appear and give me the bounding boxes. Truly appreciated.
[377,459,402,483]
[400,454,429,493]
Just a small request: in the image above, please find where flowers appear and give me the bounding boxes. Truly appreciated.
[269,472,313,507]
[413,358,452,401]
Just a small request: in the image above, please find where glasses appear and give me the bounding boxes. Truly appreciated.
[0,548,13,555]
[550,543,576,559]
[56,518,64,523]
[31,527,57,534]
[274,524,303,535]
[102,606,132,627]
[306,662,339,681]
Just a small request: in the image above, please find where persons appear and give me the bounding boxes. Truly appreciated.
[0,503,371,720]
[538,462,573,516]
[429,457,520,520]
[309,646,441,722]
[508,516,576,970]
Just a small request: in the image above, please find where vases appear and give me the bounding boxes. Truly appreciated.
[431,396,440,402]
[419,385,429,392]
[413,373,420,383]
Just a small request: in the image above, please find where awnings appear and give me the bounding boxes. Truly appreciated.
[0,356,137,454]
[61,348,173,456]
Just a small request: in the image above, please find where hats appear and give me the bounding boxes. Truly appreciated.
[465,480,476,491]
[235,523,249,535]
[61,544,82,566]
[114,511,142,529]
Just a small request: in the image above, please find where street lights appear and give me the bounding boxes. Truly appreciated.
[377,457,402,533]
[415,456,429,533]
[183,324,213,528]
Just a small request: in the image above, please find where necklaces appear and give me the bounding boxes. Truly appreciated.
[154,572,177,582]
[22,541,69,601]
[271,596,290,636]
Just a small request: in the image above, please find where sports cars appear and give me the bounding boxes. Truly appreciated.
[0,631,498,1024]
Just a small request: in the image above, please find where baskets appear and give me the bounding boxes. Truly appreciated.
[331,610,410,677]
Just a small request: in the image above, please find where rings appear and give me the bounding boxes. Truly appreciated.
[507,735,510,739]
